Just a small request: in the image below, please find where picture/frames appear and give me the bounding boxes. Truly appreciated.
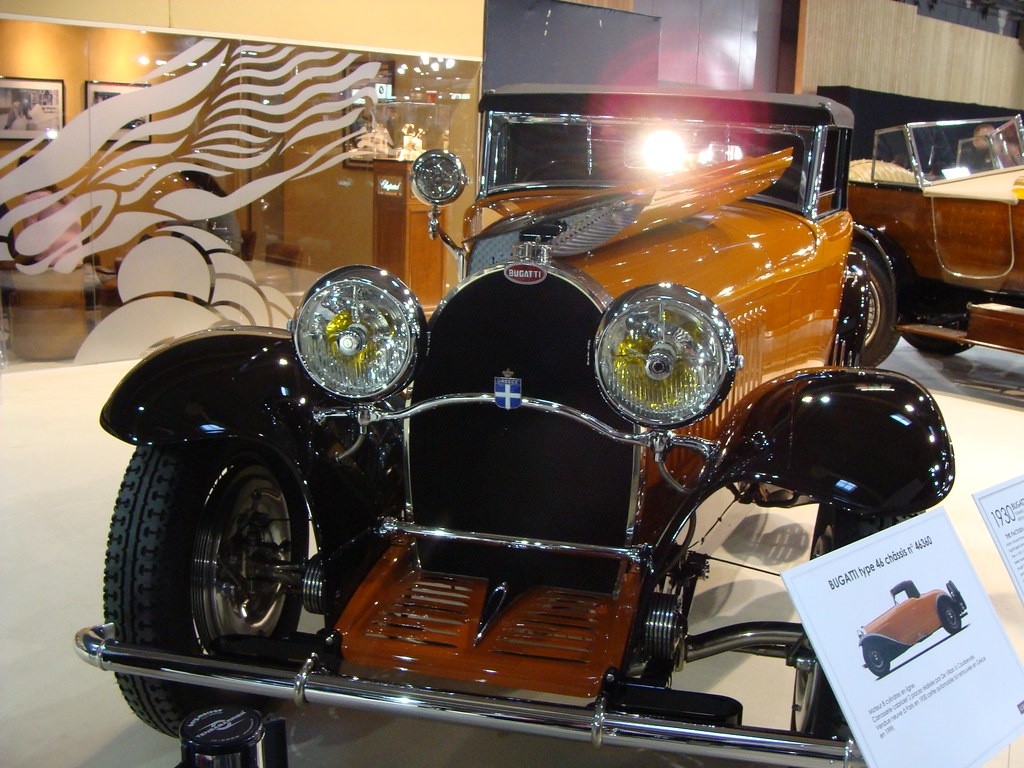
[0,76,64,142]
[85,79,152,143]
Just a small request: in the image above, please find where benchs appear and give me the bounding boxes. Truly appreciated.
[850,159,928,184]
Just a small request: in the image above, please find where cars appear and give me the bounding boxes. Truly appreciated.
[817,85,1024,368]
[72,81,958,768]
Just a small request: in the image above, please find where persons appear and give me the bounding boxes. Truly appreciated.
[969,123,1021,173]
[0,155,84,318]
[5,89,49,131]
[156,163,242,306]
